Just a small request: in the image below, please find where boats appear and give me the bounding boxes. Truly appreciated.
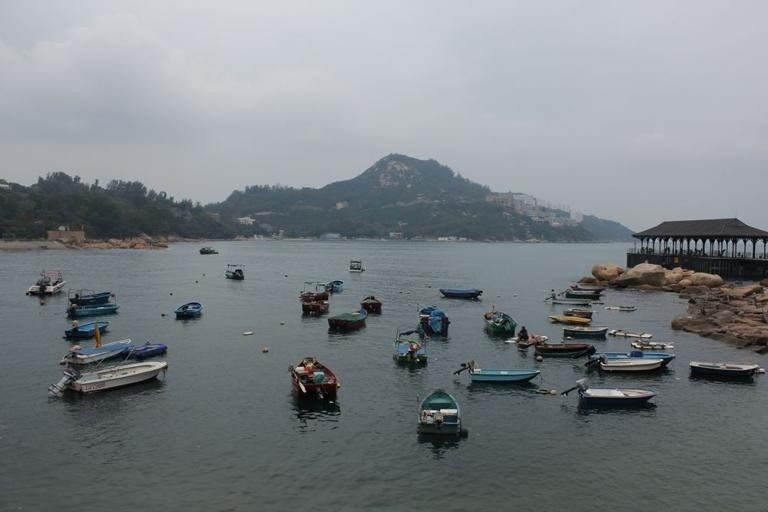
[67,302,120,319]
[28,268,67,296]
[174,302,203,320]
[689,359,765,384]
[226,267,245,280]
[69,288,113,305]
[48,321,168,400]
[200,246,219,255]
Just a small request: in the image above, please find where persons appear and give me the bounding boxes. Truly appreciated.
[71,294,81,309]
[551,290,556,300]
[71,318,79,332]
[408,342,416,360]
[517,326,529,343]
